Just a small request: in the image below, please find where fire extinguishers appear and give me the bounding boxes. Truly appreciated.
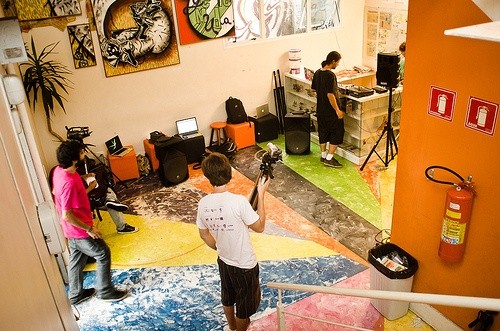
[425,165,478,262]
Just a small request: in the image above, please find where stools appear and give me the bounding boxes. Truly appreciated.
[209,122,227,147]
[143,132,205,172]
[248,113,279,144]
[107,145,140,183]
[223,122,256,150]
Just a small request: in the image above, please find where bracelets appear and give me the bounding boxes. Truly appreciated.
[89,227,92,232]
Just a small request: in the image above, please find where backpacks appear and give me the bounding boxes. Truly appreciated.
[226,97,251,127]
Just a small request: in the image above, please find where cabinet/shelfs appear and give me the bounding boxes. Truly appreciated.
[284,72,403,166]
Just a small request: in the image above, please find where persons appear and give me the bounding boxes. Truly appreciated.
[311,51,344,167]
[399,43,406,84]
[52,140,128,305]
[80,173,138,233]
[196,153,271,331]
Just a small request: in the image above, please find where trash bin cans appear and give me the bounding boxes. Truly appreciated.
[367,243,419,321]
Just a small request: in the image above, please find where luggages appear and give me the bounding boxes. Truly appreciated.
[154,134,189,187]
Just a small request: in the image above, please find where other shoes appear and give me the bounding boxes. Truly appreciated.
[321,157,326,163]
[106,200,128,212]
[70,288,96,305]
[117,223,138,233]
[324,157,342,167]
[95,288,128,302]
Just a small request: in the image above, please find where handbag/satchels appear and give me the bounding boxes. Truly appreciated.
[149,131,165,142]
[193,138,237,169]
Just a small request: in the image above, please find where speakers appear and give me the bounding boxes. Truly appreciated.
[249,113,280,143]
[173,134,206,165]
[153,137,190,187]
[284,112,310,155]
[375,52,399,88]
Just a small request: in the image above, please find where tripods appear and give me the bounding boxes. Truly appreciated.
[360,88,398,172]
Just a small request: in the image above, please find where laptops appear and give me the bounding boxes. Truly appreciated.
[105,135,132,157]
[249,103,269,119]
[176,117,203,140]
